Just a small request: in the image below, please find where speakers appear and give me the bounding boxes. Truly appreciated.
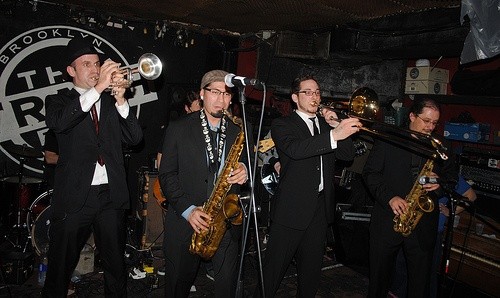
[139,170,167,248]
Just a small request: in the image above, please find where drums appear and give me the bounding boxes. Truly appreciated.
[26,189,96,259]
[0,176,43,229]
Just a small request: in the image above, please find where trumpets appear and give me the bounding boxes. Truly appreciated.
[95,53,162,96]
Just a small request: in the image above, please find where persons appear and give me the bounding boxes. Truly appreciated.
[157,68,249,298]
[437,175,477,236]
[43,39,142,298]
[257,73,362,298]
[360,98,459,298]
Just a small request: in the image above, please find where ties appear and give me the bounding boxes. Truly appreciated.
[89,104,106,166]
[309,117,322,184]
[209,128,220,172]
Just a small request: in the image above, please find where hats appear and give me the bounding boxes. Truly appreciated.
[200,70,228,88]
[62,38,104,70]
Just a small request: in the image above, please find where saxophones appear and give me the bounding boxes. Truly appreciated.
[393,131,440,237]
[189,109,245,259]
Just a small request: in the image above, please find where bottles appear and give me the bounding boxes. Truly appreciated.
[40,258,48,281]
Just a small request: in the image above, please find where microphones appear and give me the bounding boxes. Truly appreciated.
[225,74,264,87]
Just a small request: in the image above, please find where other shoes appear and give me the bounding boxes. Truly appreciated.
[206,270,215,281]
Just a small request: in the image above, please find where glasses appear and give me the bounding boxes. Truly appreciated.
[417,114,438,125]
[204,87,229,97]
[298,90,320,96]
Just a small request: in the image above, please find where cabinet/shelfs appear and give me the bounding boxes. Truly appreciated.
[408,95,500,150]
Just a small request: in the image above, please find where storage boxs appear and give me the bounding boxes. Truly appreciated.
[405,67,450,94]
[444,121,491,143]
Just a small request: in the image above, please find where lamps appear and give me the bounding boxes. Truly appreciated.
[30,0,38,12]
[65,5,108,31]
[172,28,195,48]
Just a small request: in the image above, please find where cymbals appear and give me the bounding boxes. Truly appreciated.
[13,145,43,158]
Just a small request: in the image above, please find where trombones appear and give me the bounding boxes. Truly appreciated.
[310,87,448,161]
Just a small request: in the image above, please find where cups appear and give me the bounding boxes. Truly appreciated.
[476,224,484,233]
[453,216,460,227]
